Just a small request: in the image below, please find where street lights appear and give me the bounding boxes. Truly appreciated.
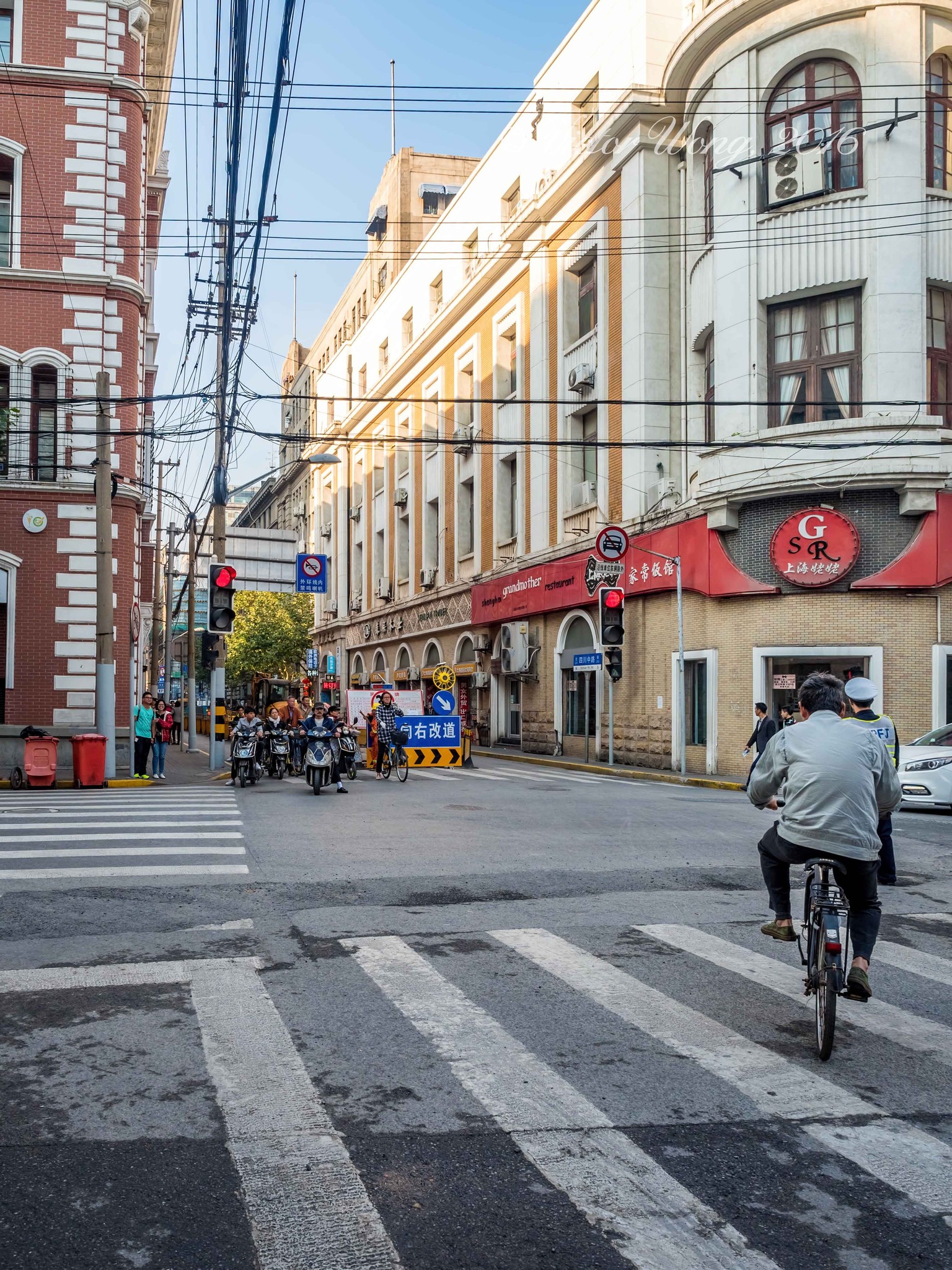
[211,452,342,769]
[164,627,205,705]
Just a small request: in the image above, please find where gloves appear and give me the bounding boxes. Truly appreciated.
[392,702,399,710]
[380,721,386,728]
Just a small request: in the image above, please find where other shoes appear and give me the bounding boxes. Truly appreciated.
[289,773,298,777]
[740,786,747,791]
[153,774,159,779]
[351,772,357,777]
[139,775,150,779]
[176,743,179,745]
[376,776,381,780]
[227,757,234,761]
[171,742,176,745]
[337,787,348,793]
[225,780,235,786]
[159,774,165,779]
[134,773,140,778]
[255,763,261,770]
[369,767,376,771]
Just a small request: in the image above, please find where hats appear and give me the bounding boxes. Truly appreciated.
[844,677,878,702]
[328,705,340,713]
[315,701,324,709]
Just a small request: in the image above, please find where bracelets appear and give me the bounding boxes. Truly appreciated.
[152,737,155,739]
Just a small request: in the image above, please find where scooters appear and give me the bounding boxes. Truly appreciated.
[228,716,309,788]
[298,719,345,796]
[329,717,363,781]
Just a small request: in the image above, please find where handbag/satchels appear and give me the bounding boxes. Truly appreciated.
[391,731,409,744]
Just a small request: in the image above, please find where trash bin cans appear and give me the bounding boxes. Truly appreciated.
[70,734,109,788]
[23,736,59,789]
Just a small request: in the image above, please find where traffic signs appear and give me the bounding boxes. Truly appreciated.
[157,689,164,694]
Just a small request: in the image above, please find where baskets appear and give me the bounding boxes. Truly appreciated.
[390,730,408,745]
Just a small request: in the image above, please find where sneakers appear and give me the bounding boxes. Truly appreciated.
[846,966,872,997]
[761,919,796,942]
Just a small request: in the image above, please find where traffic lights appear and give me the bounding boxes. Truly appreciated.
[843,666,864,681]
[605,649,623,678]
[202,632,220,668]
[599,587,625,647]
[207,562,237,634]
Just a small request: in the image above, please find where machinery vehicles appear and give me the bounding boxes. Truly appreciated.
[176,682,198,705]
[226,671,292,720]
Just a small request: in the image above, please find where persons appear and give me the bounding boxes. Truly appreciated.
[740,702,778,795]
[150,697,181,745]
[134,692,156,779]
[364,702,381,771]
[781,705,796,729]
[840,677,899,885]
[151,699,174,779]
[375,692,404,780]
[746,672,903,997]
[225,688,357,785]
[299,701,349,793]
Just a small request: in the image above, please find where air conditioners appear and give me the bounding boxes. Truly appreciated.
[358,672,369,685]
[292,425,471,612]
[768,143,825,210]
[572,480,598,509]
[647,477,677,514]
[472,672,487,688]
[406,667,419,680]
[567,363,589,392]
[501,621,529,673]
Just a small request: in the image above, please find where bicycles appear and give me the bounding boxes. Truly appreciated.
[381,723,410,782]
[772,799,869,1061]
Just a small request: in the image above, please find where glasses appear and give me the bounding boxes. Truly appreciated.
[383,696,390,699]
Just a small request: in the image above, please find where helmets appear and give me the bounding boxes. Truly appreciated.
[372,703,381,710]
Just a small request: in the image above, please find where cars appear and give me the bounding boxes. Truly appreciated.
[895,722,952,810]
